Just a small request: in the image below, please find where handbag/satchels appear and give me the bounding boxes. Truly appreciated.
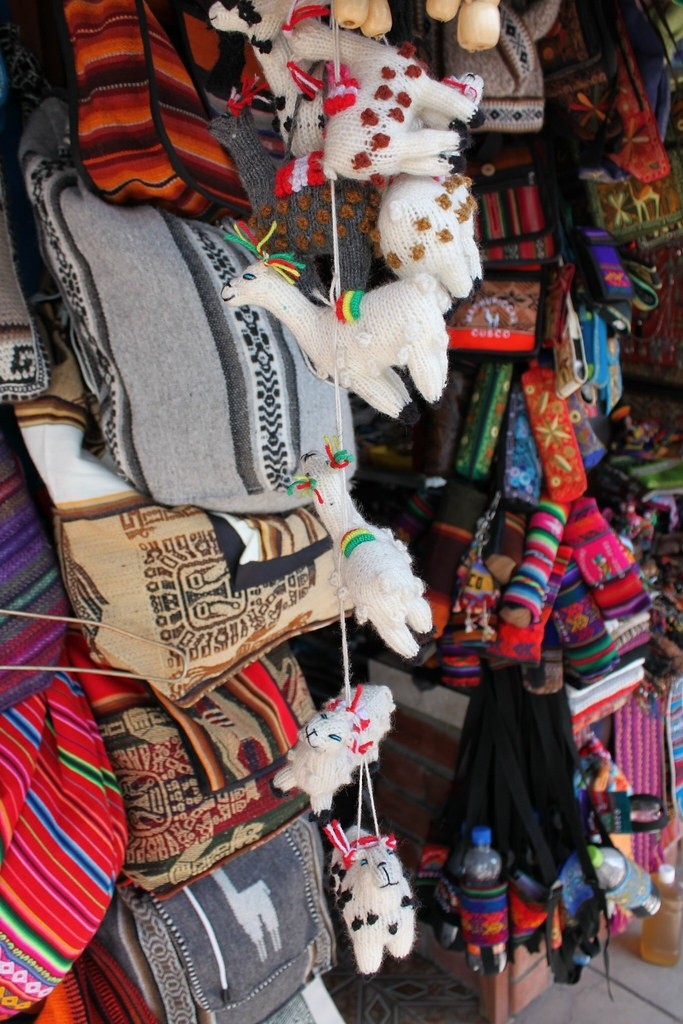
[0,1,661,1024]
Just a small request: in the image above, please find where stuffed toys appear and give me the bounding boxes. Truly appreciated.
[268,684,415,974]
[286,436,432,659]
[208,0,485,419]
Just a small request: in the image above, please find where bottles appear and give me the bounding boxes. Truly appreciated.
[462,827,509,974]
[587,846,662,918]
[640,864,683,966]
